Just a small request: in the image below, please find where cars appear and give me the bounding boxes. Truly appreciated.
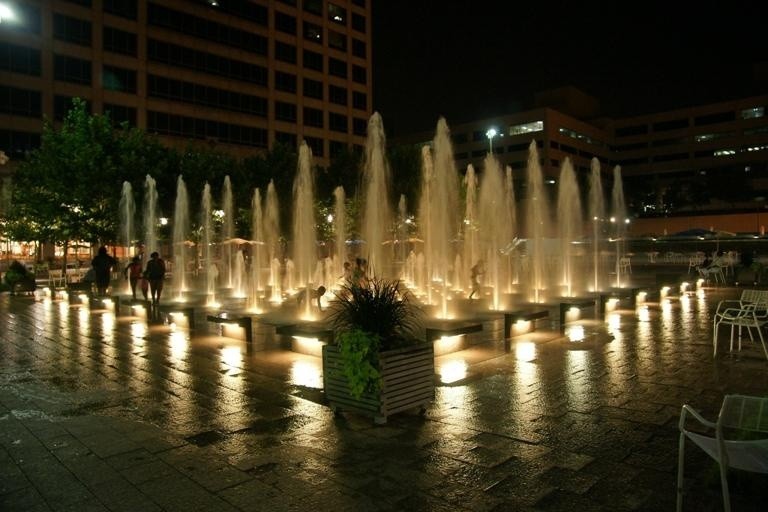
[48,263,90,277]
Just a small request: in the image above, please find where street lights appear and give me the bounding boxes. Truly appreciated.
[485,128,499,157]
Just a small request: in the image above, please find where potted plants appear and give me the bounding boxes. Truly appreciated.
[321,280,434,426]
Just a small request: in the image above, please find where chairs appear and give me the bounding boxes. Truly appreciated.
[711,289,768,358]
[48,268,175,289]
[612,247,740,285]
[676,393,767,512]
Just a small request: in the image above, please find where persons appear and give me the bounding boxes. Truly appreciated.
[297,286,326,313]
[92,246,116,296]
[145,252,166,305]
[339,258,375,288]
[138,272,148,300]
[124,256,142,298]
[469,259,488,299]
[705,250,724,284]
